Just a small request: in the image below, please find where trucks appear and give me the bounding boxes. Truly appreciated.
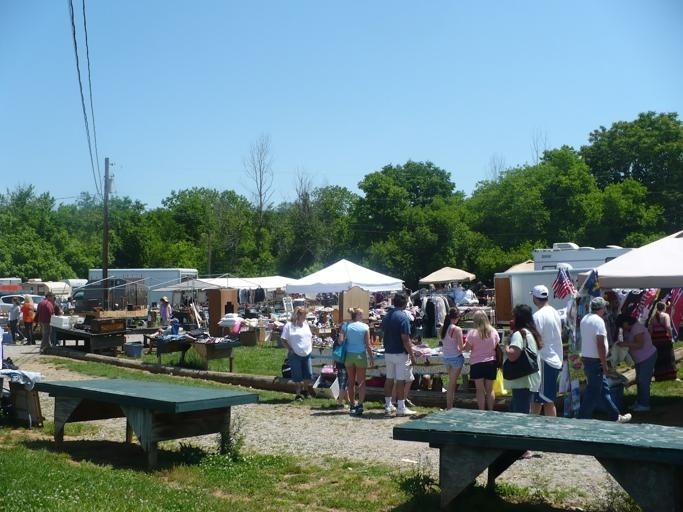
[62,277,148,312]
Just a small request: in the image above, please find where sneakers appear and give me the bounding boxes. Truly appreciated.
[616,412,632,423]
[396,408,417,417]
[629,399,652,413]
[295,392,311,402]
[385,405,397,415]
[349,402,364,414]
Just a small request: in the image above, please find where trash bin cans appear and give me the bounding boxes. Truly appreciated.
[124,342,142,358]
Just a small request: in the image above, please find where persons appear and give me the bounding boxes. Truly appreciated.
[615,313,657,411]
[143,296,196,355]
[529,285,563,416]
[580,297,632,422]
[651,302,672,381]
[503,304,542,412]
[440,306,465,408]
[332,322,350,406]
[7,292,74,350]
[465,310,501,410]
[376,290,417,416]
[280,306,317,402]
[338,307,373,414]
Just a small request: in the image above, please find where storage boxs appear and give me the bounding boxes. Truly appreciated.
[93,318,127,332]
[312,374,339,400]
[240,331,257,346]
[49,315,85,329]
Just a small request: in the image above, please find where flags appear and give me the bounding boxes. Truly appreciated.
[551,266,602,300]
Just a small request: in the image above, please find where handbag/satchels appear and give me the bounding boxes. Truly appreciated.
[503,329,539,381]
[332,323,349,364]
[495,342,504,369]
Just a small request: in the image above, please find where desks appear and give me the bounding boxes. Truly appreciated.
[53,328,132,352]
[35,377,258,470]
[312,346,471,393]
[393,409,683,512]
[155,340,241,371]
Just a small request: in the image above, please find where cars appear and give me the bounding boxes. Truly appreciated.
[475,287,495,302]
[0,294,53,315]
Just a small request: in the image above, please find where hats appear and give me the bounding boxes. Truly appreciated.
[590,296,610,310]
[529,284,549,300]
[161,296,169,302]
